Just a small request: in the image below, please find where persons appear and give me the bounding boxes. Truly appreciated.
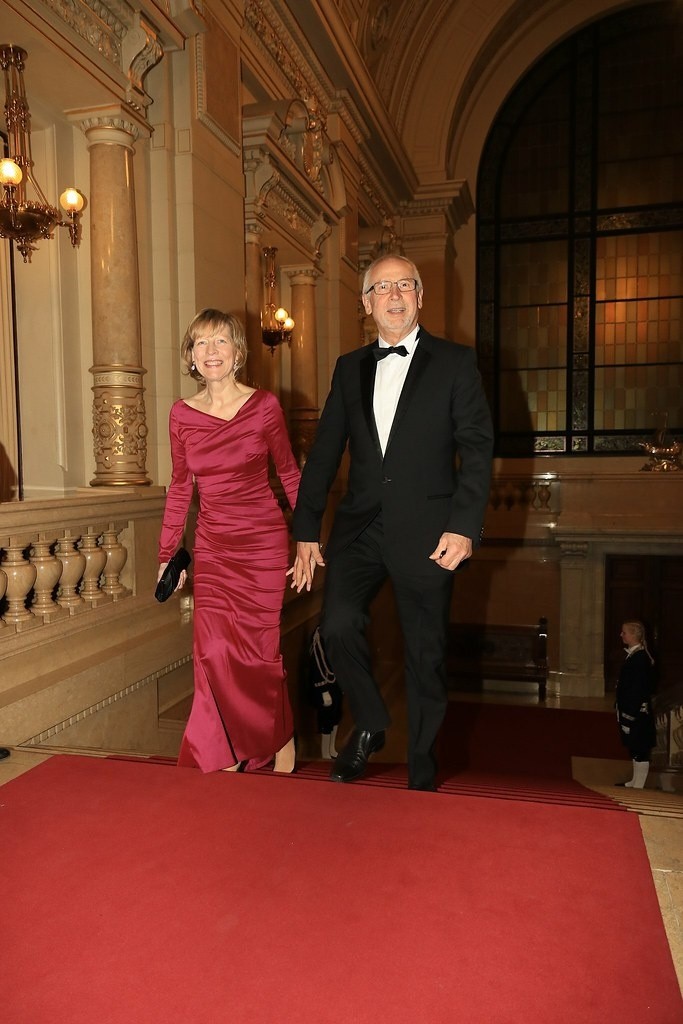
[149,307,315,773]
[614,621,656,788]
[303,629,343,760]
[292,255,492,794]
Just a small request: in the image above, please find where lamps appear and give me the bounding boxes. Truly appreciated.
[0,42,85,262]
[257,246,295,355]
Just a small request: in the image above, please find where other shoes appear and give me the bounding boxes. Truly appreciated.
[221,760,245,772]
[273,747,297,773]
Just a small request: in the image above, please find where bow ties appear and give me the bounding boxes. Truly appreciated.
[372,346,409,361]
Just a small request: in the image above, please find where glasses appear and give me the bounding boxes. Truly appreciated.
[366,279,418,295]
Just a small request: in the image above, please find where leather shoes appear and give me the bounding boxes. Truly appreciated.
[330,728,387,782]
[408,782,434,792]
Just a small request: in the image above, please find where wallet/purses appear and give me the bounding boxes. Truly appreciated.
[155,547,191,602]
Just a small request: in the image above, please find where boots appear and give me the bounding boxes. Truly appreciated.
[329,725,339,758]
[633,760,650,789]
[624,760,638,787]
[321,733,332,759]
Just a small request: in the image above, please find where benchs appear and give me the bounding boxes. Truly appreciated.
[445,615,549,703]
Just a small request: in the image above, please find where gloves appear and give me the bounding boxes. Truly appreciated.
[622,725,630,734]
[322,691,332,706]
